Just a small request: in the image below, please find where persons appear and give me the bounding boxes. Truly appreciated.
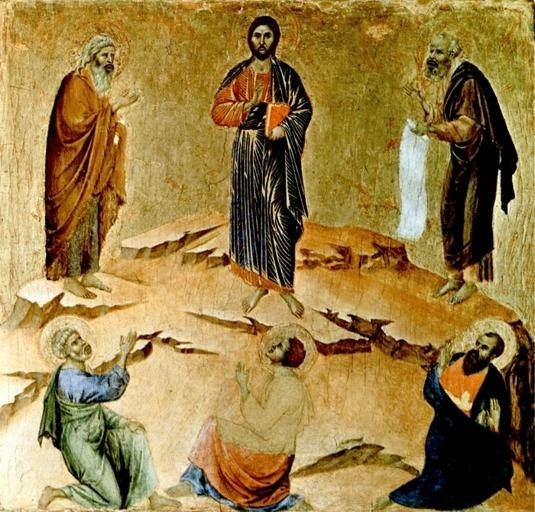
[410,34,523,307]
[212,13,321,319]
[168,321,334,512]
[44,34,139,299]
[30,313,183,512]
[390,319,518,512]
